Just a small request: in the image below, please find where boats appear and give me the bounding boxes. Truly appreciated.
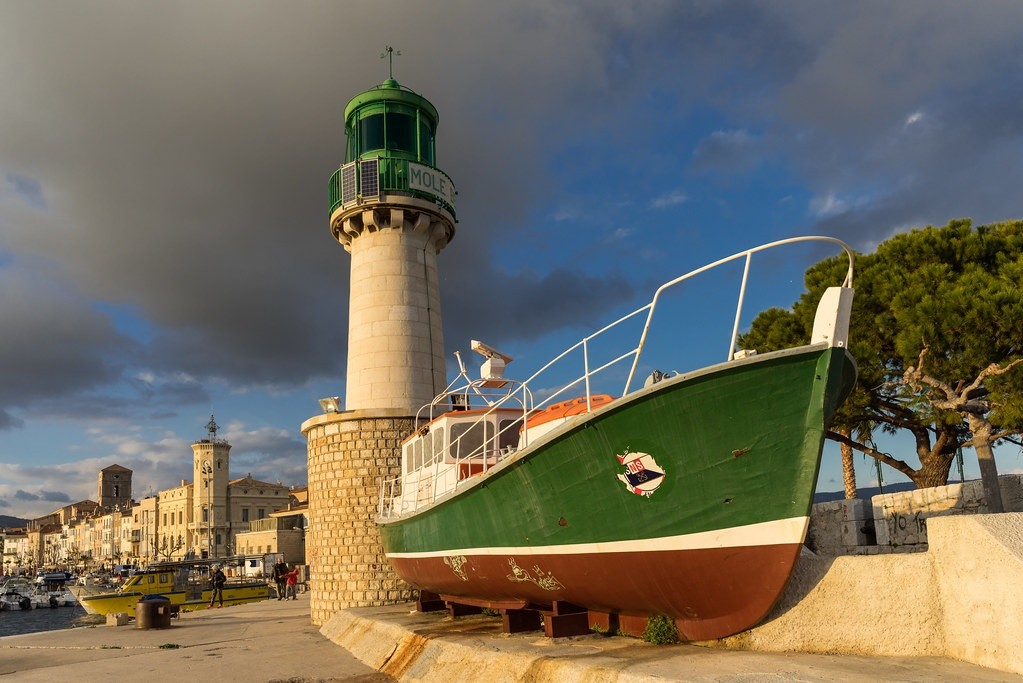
[371,236,856,645]
[517,394,615,449]
[0,565,128,614]
[82,554,270,620]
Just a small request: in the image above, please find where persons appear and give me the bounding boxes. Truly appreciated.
[206,565,227,609]
[272,558,299,601]
[305,565,310,591]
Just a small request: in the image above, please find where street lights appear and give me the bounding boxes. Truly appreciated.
[142,510,148,566]
[202,466,212,558]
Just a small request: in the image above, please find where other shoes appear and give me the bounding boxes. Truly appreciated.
[207,604,213,609]
[278,597,282,601]
[217,604,222,608]
[285,597,289,601]
[293,597,298,600]
